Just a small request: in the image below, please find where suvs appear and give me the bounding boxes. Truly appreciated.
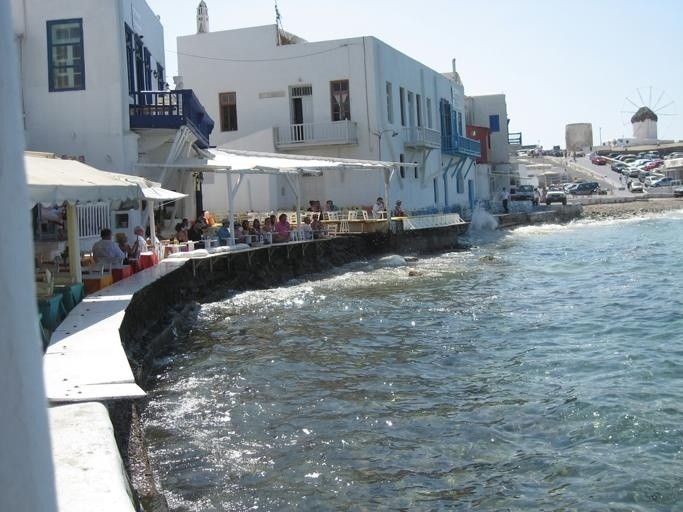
[545,184,568,205]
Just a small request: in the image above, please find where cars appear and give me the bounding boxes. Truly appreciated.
[523,144,683,197]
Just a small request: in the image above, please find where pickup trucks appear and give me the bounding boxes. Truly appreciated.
[509,183,540,205]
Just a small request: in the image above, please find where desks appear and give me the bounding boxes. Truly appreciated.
[377,211,387,219]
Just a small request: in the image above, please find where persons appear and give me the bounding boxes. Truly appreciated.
[187,220,202,240]
[143,224,160,244]
[173,222,186,241]
[276,213,290,237]
[216,219,231,245]
[261,217,273,233]
[305,199,322,212]
[90,228,125,261]
[270,214,277,232]
[323,200,337,211]
[392,199,404,216]
[498,187,510,214]
[303,217,312,232]
[196,209,215,226]
[372,196,388,220]
[127,225,147,250]
[541,187,548,202]
[233,220,245,242]
[532,187,540,206]
[252,219,262,234]
[115,231,139,256]
[242,219,255,235]
[311,214,324,230]
